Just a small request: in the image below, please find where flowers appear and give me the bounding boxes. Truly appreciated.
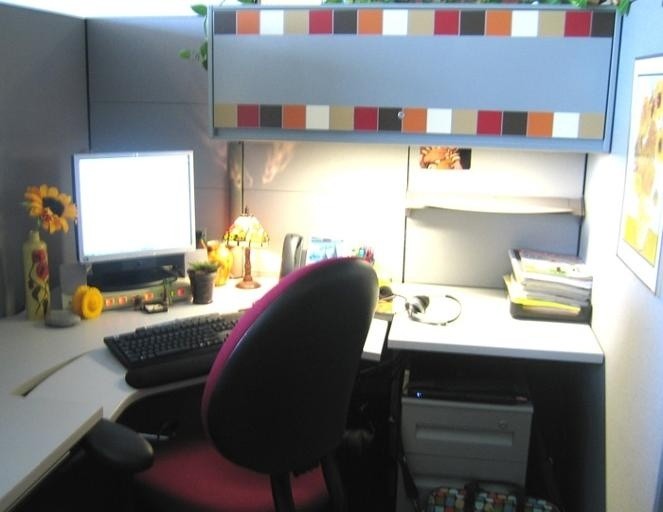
[15,184,77,235]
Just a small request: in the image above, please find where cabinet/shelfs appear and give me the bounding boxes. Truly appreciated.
[393,367,535,512]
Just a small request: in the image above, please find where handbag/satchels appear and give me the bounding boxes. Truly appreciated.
[425,481,554,512]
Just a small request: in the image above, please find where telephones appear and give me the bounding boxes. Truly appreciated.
[279,234,308,278]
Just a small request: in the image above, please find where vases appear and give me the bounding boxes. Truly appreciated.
[21,231,51,321]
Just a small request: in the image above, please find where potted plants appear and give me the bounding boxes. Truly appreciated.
[186,260,221,305]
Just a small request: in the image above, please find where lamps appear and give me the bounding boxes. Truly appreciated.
[222,206,271,289]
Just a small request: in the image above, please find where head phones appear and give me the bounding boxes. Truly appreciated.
[405,294,463,326]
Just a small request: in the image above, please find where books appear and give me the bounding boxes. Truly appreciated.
[502,248,593,323]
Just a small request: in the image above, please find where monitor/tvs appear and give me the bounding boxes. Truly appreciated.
[63,148,197,264]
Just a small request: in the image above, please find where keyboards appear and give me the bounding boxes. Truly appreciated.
[104,309,249,369]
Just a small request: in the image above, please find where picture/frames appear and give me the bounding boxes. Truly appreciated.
[615,53,663,296]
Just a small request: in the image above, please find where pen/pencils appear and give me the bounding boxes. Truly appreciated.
[357,247,374,262]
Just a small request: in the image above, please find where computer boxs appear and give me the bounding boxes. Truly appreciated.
[59,248,208,312]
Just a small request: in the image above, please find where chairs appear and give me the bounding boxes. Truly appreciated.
[126,255,380,511]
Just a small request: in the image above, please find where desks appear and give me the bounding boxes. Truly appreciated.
[0,276,390,512]
[387,281,605,365]
